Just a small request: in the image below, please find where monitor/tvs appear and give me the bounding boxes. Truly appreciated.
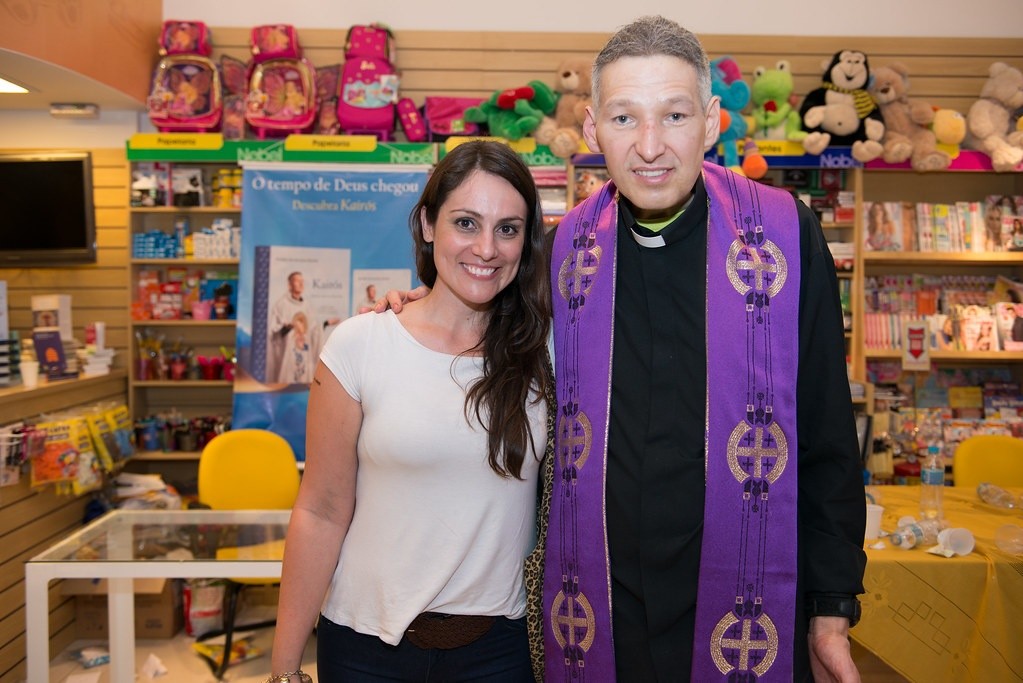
[0,153,95,266]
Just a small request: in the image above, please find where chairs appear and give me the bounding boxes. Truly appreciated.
[951,436,1023,488]
[186,429,302,678]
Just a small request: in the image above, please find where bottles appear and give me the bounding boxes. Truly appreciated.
[890,519,949,550]
[976,480,1015,509]
[919,445,944,520]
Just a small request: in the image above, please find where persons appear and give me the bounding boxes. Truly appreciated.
[358,17,874,683]
[986,203,1009,252]
[935,318,956,352]
[1007,306,1023,342]
[977,324,996,352]
[265,141,559,683]
[270,269,339,383]
[865,201,900,251]
[359,284,381,314]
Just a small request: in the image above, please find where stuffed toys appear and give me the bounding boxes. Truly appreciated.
[797,46,885,163]
[746,58,819,148]
[962,60,1023,172]
[703,53,767,179]
[462,79,559,143]
[531,55,595,157]
[864,60,967,172]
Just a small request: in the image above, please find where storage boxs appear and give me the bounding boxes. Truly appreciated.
[61,578,183,638]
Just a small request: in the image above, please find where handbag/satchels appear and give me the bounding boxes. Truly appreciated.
[178,579,225,638]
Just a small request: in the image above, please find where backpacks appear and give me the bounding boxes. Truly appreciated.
[148,18,487,144]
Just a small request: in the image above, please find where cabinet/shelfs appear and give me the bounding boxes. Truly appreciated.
[127,133,1023,466]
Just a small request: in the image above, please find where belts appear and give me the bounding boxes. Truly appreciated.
[405,612,497,649]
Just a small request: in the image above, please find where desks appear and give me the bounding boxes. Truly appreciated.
[23,510,293,683]
[848,484,1023,683]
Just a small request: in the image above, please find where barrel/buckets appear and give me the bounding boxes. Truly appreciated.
[864,492,885,540]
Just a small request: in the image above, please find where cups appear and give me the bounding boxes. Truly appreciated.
[995,524,1023,554]
[18,361,39,388]
[937,527,975,556]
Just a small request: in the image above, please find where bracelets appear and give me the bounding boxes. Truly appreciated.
[265,666,313,683]
